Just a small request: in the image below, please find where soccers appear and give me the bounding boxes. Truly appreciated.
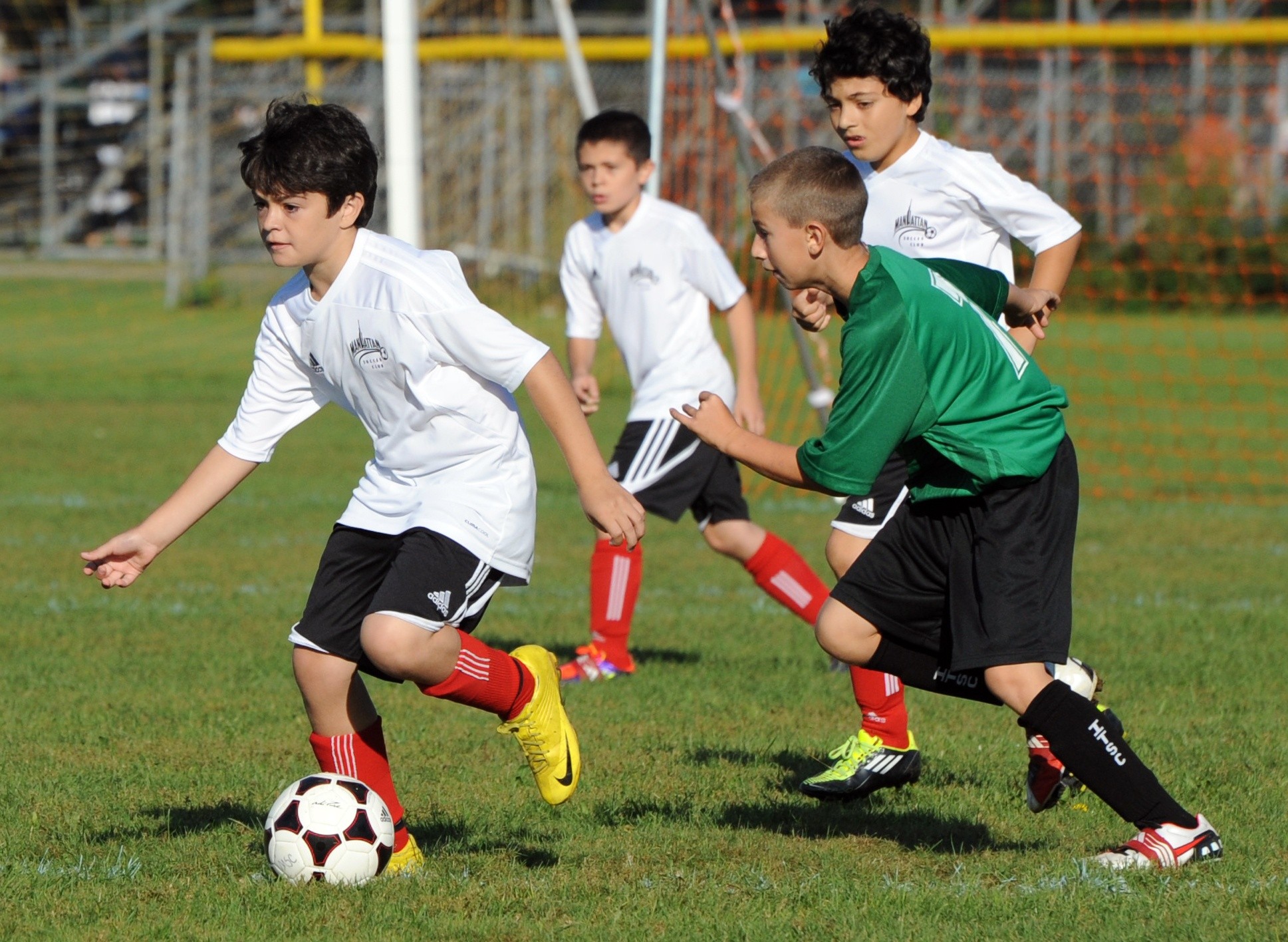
[263,773,394,887]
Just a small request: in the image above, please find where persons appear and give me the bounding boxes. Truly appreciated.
[670,145,1227,876]
[558,108,832,686]
[77,99,647,884]
[800,4,1133,816]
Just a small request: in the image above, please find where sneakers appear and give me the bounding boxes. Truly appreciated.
[496,644,582,806]
[1053,700,1128,806]
[1017,657,1106,814]
[379,833,426,879]
[1078,813,1224,870]
[802,726,920,798]
[558,645,634,684]
